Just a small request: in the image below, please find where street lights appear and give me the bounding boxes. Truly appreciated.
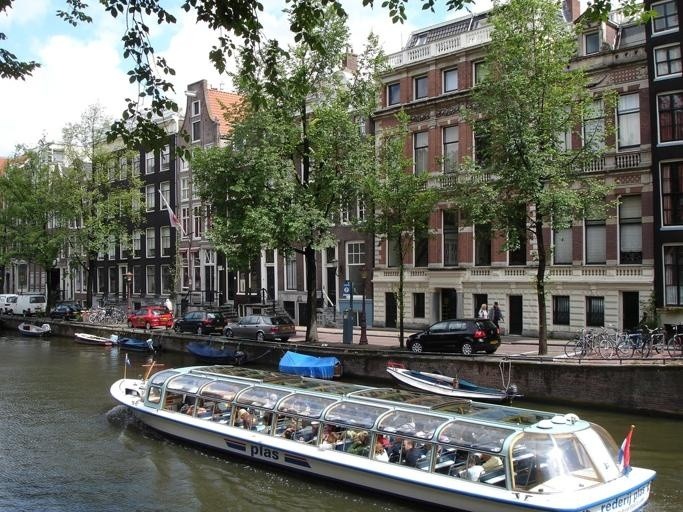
[123,272,133,315]
[358,268,369,346]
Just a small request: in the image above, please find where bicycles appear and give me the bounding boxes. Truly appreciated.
[564,324,683,360]
[81,306,126,325]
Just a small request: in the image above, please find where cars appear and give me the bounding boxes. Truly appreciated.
[173,310,229,336]
[405,317,502,356]
[126,306,174,329]
[0,293,18,315]
[49,305,83,322]
[222,314,296,342]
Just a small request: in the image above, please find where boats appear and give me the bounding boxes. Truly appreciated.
[116,337,158,352]
[18,322,51,337]
[280,354,344,377]
[117,337,154,352]
[185,343,247,363]
[17,322,45,336]
[186,342,245,365]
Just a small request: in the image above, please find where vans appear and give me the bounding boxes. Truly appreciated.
[7,293,48,318]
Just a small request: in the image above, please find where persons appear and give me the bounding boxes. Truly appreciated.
[487,302,505,328]
[479,304,487,319]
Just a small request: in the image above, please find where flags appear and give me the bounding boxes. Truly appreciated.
[158,190,180,228]
[616,424,633,476]
[205,217,212,226]
